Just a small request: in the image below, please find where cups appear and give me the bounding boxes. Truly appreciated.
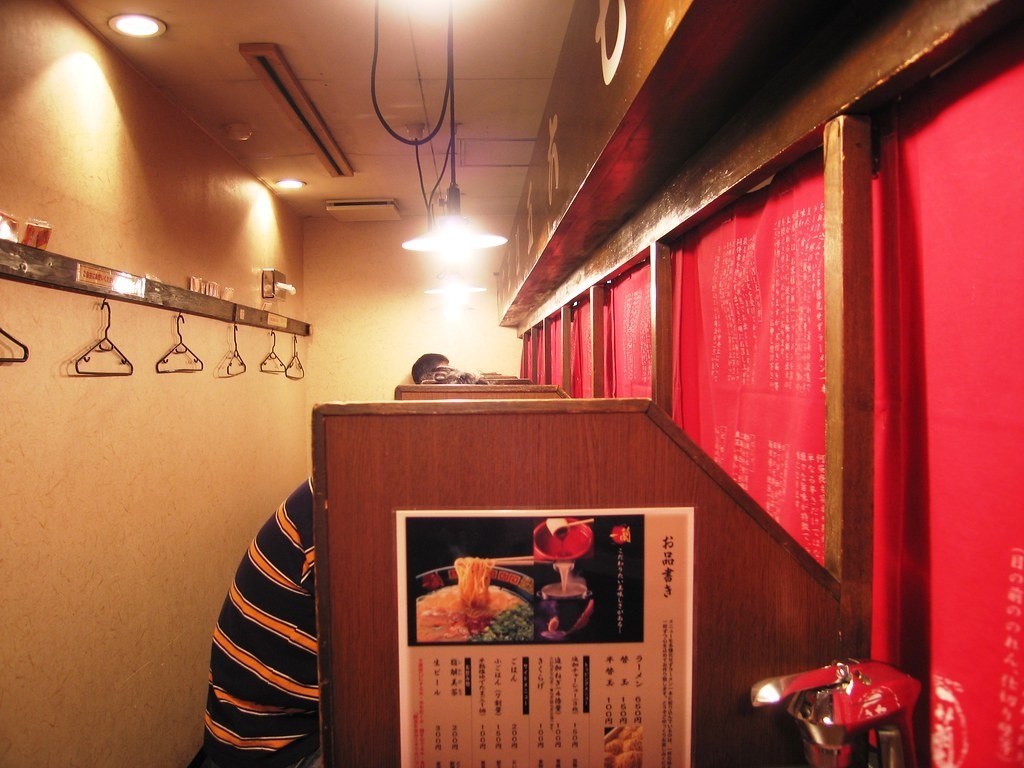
[537,582,594,631]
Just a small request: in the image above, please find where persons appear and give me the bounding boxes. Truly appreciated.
[411,354,449,384]
[202,476,322,768]
[421,366,490,385]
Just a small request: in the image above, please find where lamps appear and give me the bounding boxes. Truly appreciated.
[402,2,508,251]
[424,273,487,293]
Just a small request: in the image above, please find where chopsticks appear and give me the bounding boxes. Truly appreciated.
[454,555,534,566]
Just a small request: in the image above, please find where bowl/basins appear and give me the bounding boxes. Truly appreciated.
[532,517,594,563]
[415,566,533,642]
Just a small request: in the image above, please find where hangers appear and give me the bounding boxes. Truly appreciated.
[75,302,133,376]
[260,332,286,372]
[156,315,203,373]
[285,336,304,379]
[227,326,246,375]
[0,327,29,362]
